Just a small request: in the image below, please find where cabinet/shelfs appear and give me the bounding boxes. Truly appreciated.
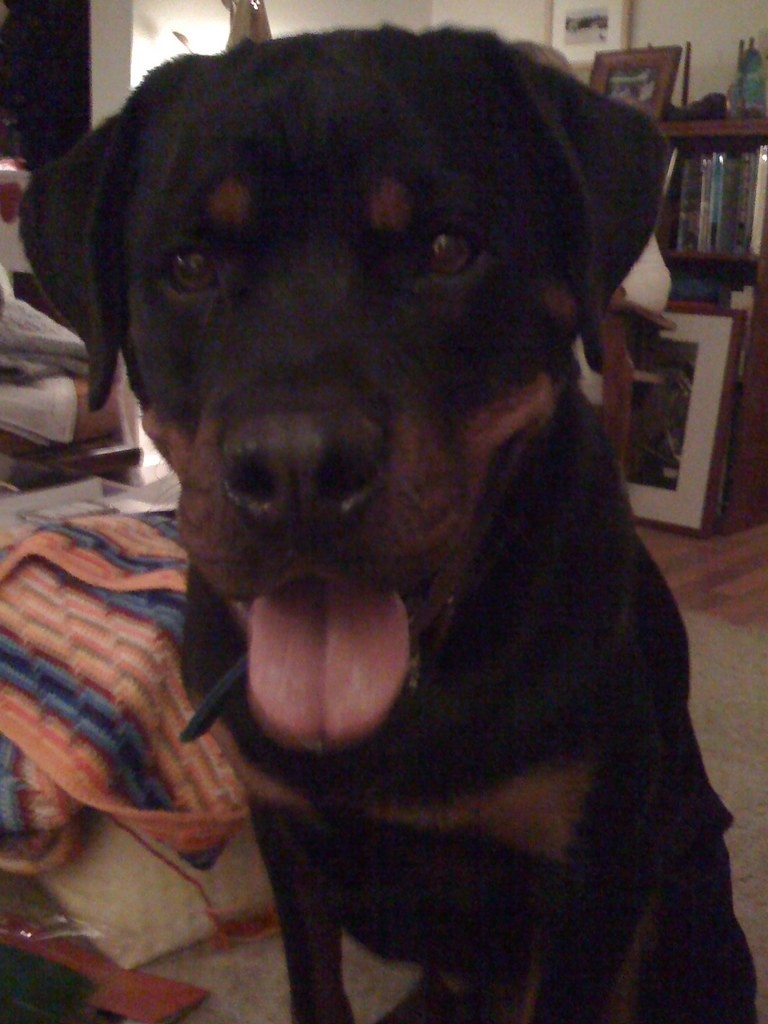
[626,124,768,530]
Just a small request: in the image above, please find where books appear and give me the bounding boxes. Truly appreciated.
[655,145,767,254]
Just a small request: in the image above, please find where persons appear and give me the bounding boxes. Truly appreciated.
[611,74,656,102]
[513,38,671,406]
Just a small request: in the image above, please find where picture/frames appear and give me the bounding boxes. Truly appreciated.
[548,0,631,70]
[592,46,681,124]
[584,298,747,538]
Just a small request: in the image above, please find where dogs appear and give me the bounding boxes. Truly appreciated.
[25,27,759,1024]
